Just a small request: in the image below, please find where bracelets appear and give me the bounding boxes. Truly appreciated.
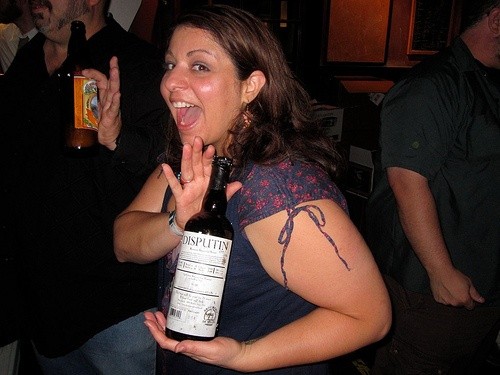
[169,209,184,237]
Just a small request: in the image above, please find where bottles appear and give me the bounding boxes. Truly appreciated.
[60,20,100,149]
[164,156,235,341]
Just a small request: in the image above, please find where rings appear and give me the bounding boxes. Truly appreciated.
[180,173,194,183]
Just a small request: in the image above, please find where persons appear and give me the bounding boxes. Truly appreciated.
[381,0,500,375]
[0,0,169,375]
[113,4,394,375]
